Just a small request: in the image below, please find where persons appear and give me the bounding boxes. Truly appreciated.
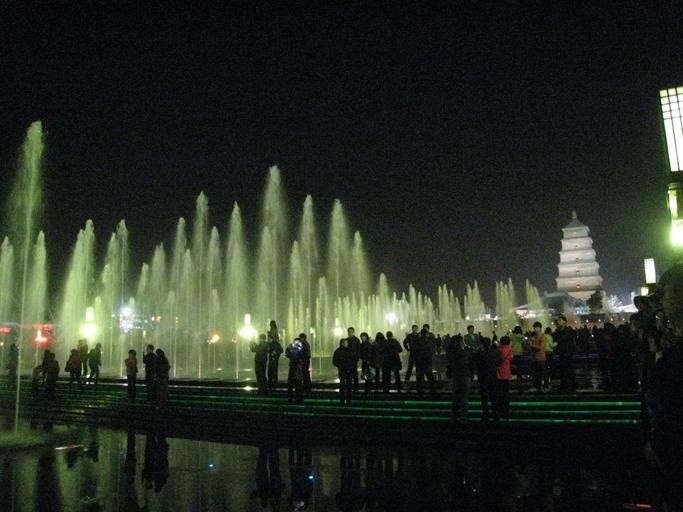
[286,333,312,405]
[251,322,283,395]
[629,266,681,511]
[125,349,137,399]
[32,338,101,401]
[6,344,18,390]
[142,345,157,402]
[332,316,629,405]
[445,335,513,424]
[156,348,169,402]
